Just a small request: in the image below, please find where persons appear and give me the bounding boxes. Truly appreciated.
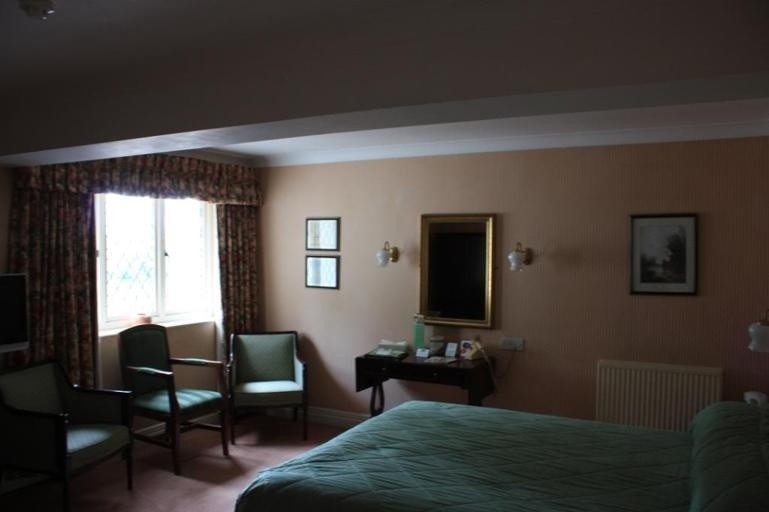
[460,342,474,360]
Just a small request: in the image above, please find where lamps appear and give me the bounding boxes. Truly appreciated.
[376,241,398,266]
[507,242,531,268]
[748,310,769,353]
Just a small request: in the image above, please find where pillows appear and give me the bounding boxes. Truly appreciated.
[688,401,769,512]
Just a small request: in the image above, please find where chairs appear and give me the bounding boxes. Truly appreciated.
[0,359,133,511]
[118,324,228,474]
[226,330,308,444]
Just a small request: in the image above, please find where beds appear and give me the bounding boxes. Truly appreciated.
[234,399,769,512]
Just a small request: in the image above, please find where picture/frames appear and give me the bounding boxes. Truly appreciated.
[419,213,496,329]
[306,218,339,251]
[305,255,339,289]
[626,213,698,296]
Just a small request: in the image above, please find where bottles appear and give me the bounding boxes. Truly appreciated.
[414,316,424,351]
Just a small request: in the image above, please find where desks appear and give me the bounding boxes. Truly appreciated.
[355,346,497,417]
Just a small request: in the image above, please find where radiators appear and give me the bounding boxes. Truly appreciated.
[596,358,724,431]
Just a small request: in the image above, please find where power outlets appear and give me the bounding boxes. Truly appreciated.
[497,337,523,350]
